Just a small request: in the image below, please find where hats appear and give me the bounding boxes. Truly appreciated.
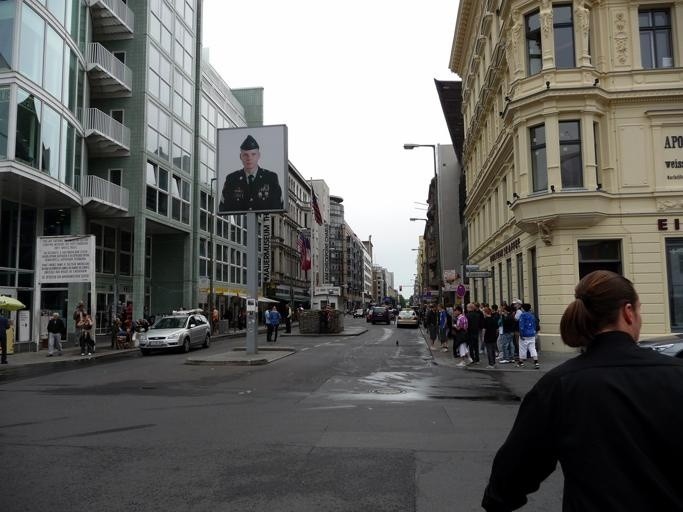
[77,301,86,309]
[240,134,259,150]
[512,298,522,305]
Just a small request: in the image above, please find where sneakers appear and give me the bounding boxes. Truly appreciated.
[532,362,540,369]
[512,362,527,369]
[440,344,516,369]
[80,352,92,357]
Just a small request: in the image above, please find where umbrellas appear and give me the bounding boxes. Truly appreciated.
[0,295,26,317]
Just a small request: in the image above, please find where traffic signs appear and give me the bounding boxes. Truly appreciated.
[465,264,481,272]
[465,272,492,279]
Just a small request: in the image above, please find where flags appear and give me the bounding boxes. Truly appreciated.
[296,237,304,248]
[301,240,312,269]
[312,185,323,226]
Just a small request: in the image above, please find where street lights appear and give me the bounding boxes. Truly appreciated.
[322,247,338,285]
[290,228,309,322]
[408,216,429,292]
[409,247,425,305]
[402,142,445,308]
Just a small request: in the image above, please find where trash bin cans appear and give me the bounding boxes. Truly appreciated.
[219,319,229,334]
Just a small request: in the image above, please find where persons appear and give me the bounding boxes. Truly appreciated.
[318,306,328,334]
[46,313,65,357]
[219,135,284,212]
[415,299,539,369]
[0,316,10,364]
[481,270,683,512]
[73,303,304,356]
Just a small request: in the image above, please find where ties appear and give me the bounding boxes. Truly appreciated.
[248,174,254,184]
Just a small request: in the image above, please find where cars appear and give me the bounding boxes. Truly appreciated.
[635,333,683,360]
[348,304,420,329]
[135,308,212,358]
